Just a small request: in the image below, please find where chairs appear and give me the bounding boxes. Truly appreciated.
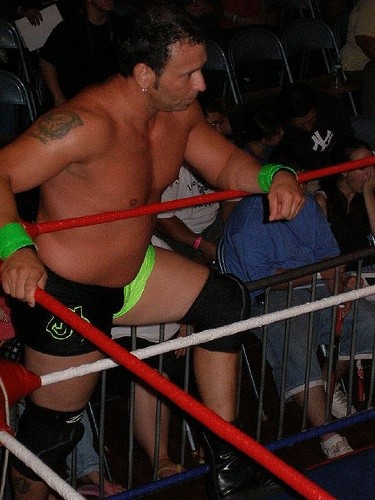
[0,17,358,150]
